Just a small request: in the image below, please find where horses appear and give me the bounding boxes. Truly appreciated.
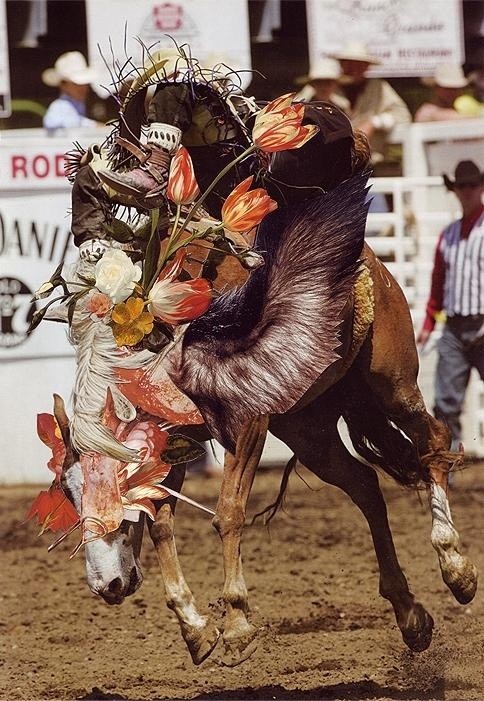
[58,214,477,669]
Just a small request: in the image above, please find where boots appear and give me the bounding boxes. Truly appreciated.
[97,148,172,210]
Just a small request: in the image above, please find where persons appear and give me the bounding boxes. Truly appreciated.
[41,49,107,129]
[412,61,484,125]
[294,37,411,142]
[416,159,484,491]
[96,80,354,211]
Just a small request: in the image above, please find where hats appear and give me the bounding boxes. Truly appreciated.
[442,159,484,191]
[319,35,381,71]
[431,56,470,91]
[41,49,98,88]
